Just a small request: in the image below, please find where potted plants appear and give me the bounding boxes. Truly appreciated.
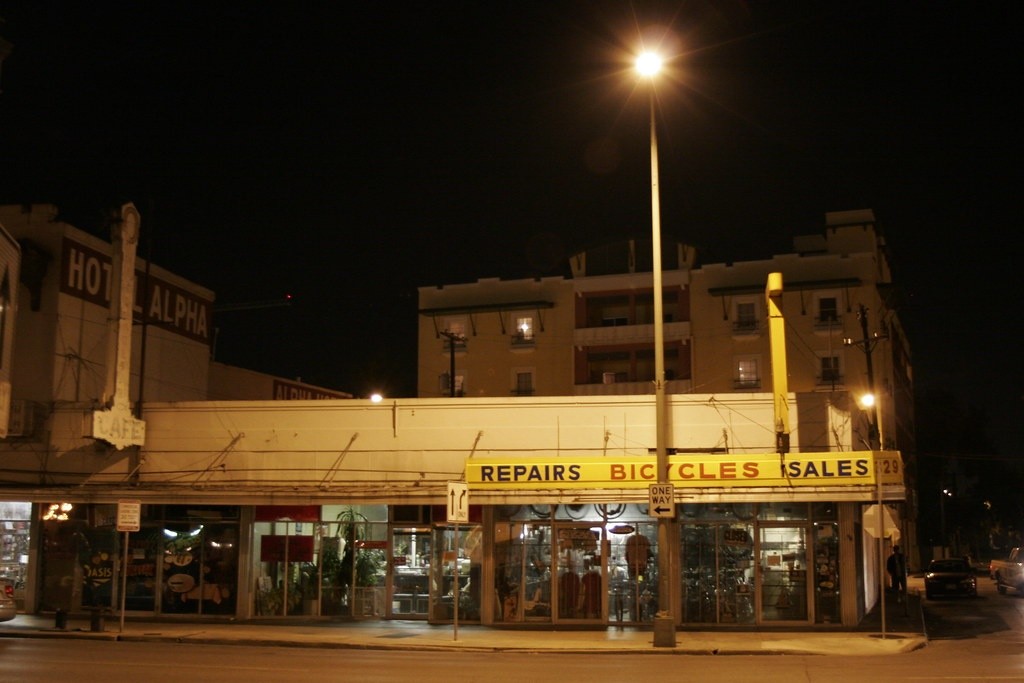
[347,551,386,616]
[300,563,319,615]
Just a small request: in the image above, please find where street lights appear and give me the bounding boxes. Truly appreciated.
[635,48,670,512]
[940,481,953,554]
[860,390,888,642]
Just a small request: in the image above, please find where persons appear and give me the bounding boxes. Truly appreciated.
[886,545,911,610]
[447,577,471,610]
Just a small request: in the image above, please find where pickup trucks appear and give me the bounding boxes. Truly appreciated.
[989,547,1024,595]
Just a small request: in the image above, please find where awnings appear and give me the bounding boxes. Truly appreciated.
[417,300,554,338]
[876,282,909,323]
[708,276,862,321]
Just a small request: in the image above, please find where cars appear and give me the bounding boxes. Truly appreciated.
[968,545,996,572]
[921,558,977,599]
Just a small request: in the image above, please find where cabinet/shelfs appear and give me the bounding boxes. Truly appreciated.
[0,520,31,600]
[393,565,470,613]
[508,539,657,622]
[681,523,841,623]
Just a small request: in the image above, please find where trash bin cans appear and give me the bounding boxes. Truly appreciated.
[91,606,105,631]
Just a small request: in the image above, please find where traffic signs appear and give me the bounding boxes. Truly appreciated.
[647,483,676,519]
